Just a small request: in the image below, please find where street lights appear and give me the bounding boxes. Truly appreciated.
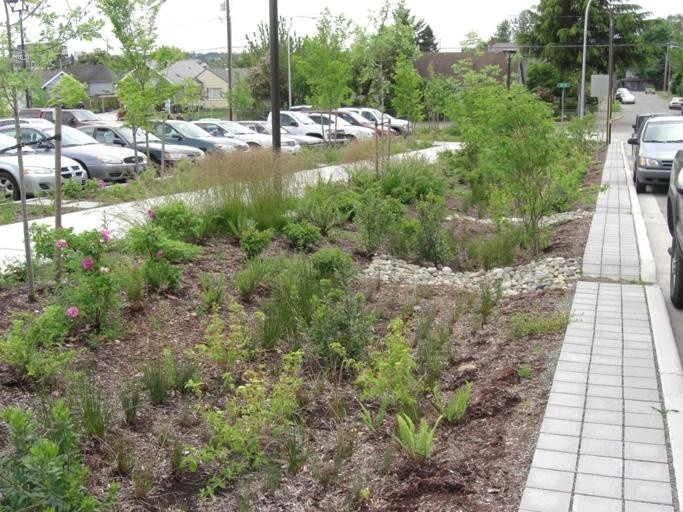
[285,15,319,110]
[501,48,517,111]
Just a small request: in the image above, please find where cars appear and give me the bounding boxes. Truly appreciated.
[236,120,327,149]
[614,86,636,105]
[668,96,683,109]
[72,123,206,177]
[1,122,148,182]
[664,150,682,310]
[1,101,413,144]
[1,133,89,198]
[141,119,250,160]
[627,116,683,194]
[188,119,303,156]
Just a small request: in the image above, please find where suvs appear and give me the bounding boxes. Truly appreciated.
[630,111,670,159]
[644,83,655,95]
